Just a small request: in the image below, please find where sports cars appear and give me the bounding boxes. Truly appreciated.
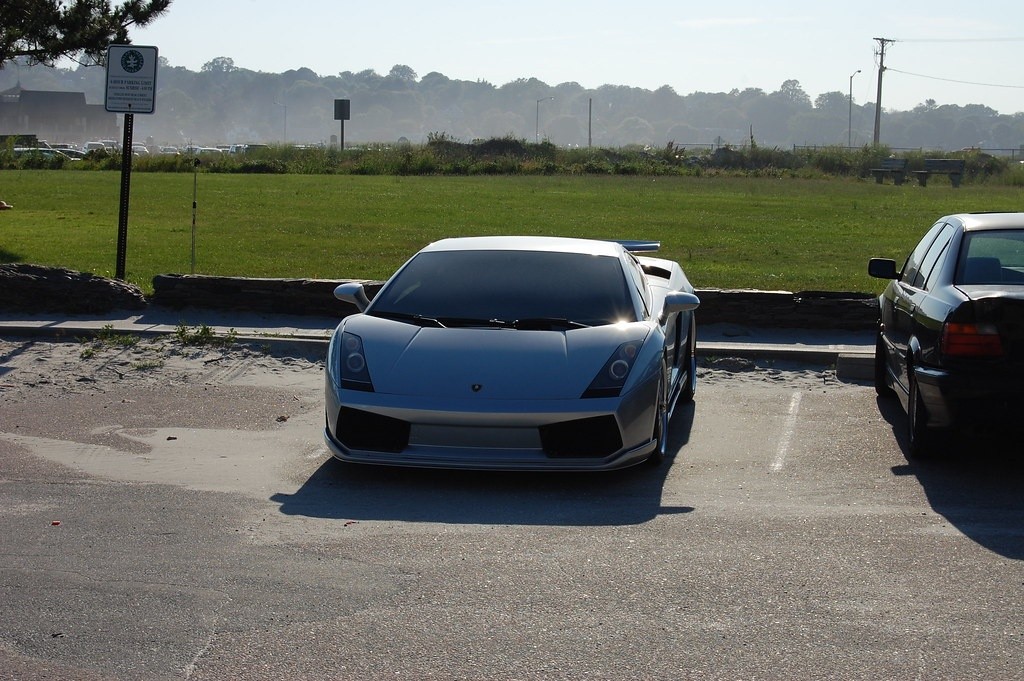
[323,237,699,488]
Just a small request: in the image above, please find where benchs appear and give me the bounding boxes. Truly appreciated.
[868,158,909,185]
[912,158,965,188]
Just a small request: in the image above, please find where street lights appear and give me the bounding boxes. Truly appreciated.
[848,70,865,148]
[273,101,287,145]
[535,96,555,141]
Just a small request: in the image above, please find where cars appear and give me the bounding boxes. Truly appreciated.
[10,138,124,162]
[867,212,1024,454]
[132,142,273,160]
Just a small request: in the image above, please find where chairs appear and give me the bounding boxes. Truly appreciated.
[960,257,1024,285]
